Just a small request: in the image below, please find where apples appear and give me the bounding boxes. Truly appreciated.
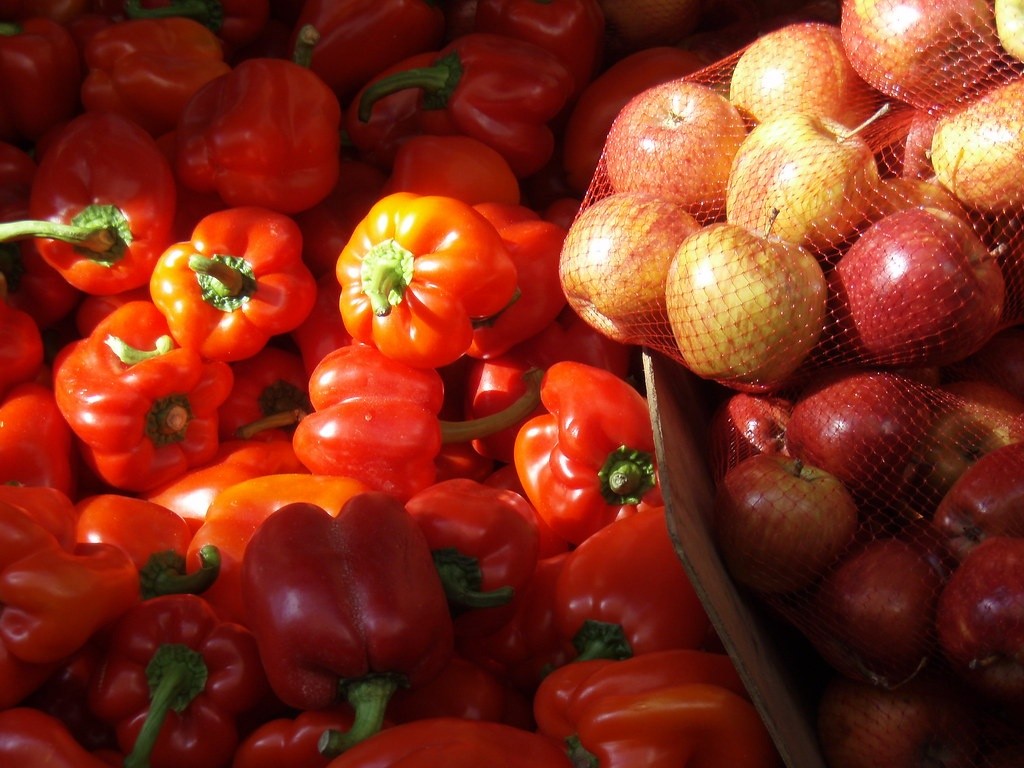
[558,1,1024,395]
[703,317,1023,768]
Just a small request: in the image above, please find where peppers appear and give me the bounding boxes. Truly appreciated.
[3,0,777,766]
